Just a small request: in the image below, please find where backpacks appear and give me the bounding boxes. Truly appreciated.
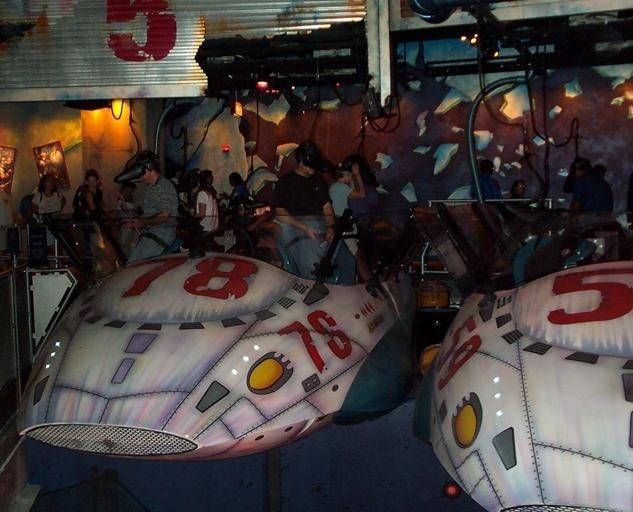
[21,191,59,225]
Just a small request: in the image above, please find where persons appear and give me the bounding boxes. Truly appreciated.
[510,179,527,198]
[445,178,476,199]
[27,175,67,222]
[74,189,104,230]
[592,163,606,180]
[561,159,607,263]
[472,159,502,198]
[0,187,13,250]
[111,141,383,284]
[72,169,103,217]
